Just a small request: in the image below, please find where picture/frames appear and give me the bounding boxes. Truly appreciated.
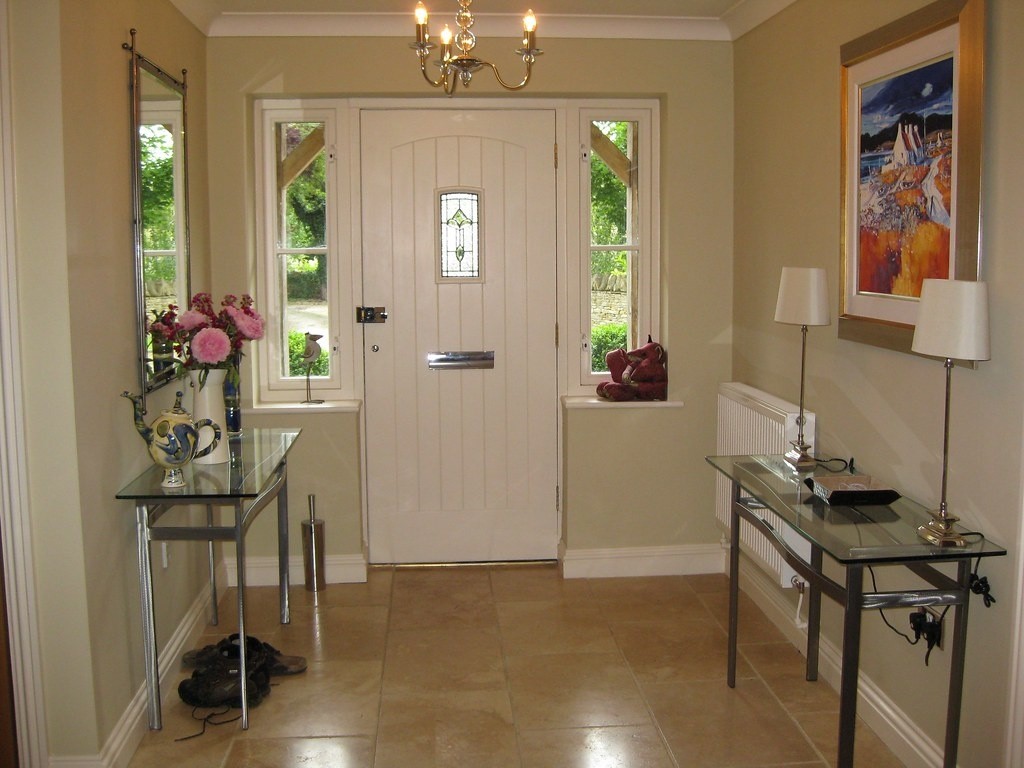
[838,0,988,372]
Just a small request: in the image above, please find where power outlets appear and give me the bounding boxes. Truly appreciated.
[917,606,945,651]
[163,542,177,567]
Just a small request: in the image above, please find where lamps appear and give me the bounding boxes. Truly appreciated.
[911,278,991,548]
[409,0,544,99]
[775,266,831,471]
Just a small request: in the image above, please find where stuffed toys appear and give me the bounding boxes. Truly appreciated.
[596,334,667,401]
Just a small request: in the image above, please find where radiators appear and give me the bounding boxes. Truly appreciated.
[714,382,815,588]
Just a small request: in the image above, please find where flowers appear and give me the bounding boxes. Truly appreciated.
[150,292,263,394]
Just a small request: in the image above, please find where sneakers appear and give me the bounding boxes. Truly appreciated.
[172,679,271,742]
[191,661,280,697]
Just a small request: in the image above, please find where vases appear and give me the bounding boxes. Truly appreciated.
[188,369,232,465]
[222,371,243,437]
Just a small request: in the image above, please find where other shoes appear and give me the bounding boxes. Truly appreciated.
[183,633,307,674]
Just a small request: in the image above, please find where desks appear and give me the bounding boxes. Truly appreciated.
[115,426,303,732]
[705,451,1007,768]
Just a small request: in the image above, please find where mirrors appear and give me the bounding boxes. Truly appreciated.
[122,28,192,399]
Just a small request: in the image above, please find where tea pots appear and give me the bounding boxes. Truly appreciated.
[120,389,222,487]
